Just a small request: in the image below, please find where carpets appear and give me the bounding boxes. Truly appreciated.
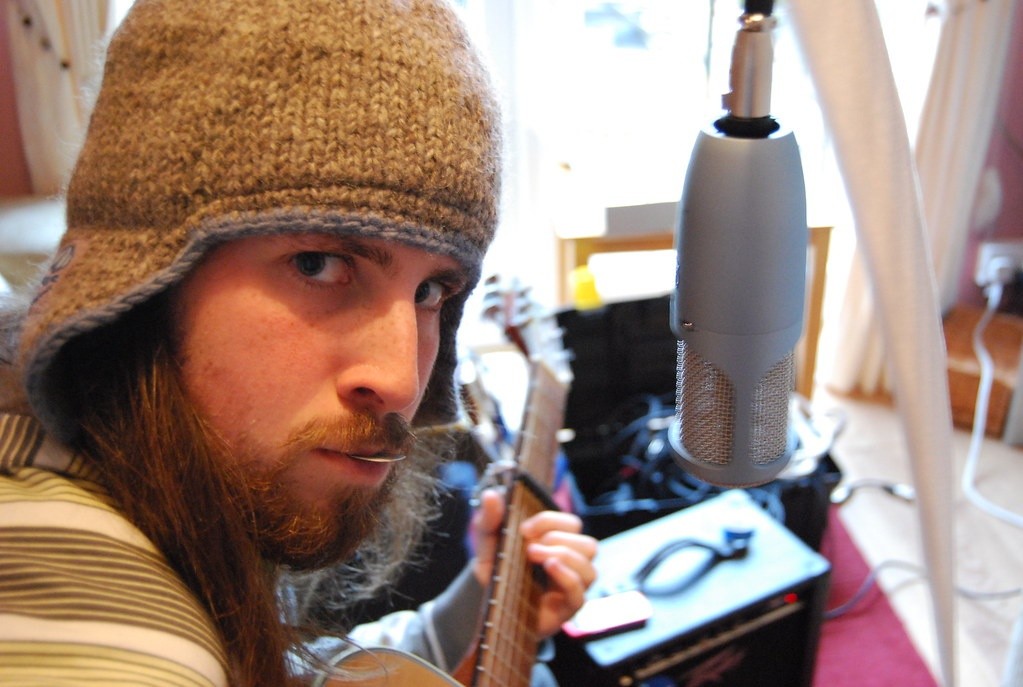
[469,480,940,687]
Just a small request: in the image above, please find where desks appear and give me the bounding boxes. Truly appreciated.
[553,220,833,412]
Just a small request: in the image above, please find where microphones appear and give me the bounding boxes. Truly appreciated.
[666,0,803,486]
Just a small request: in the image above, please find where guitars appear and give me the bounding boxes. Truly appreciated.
[284,271,576,687]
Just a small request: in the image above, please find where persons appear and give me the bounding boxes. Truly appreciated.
[0,0,597,687]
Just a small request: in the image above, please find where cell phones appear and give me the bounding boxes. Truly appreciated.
[559,589,652,639]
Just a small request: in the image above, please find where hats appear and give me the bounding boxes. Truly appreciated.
[22,0,501,440]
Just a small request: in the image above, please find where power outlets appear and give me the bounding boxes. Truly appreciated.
[971,239,1023,287]
